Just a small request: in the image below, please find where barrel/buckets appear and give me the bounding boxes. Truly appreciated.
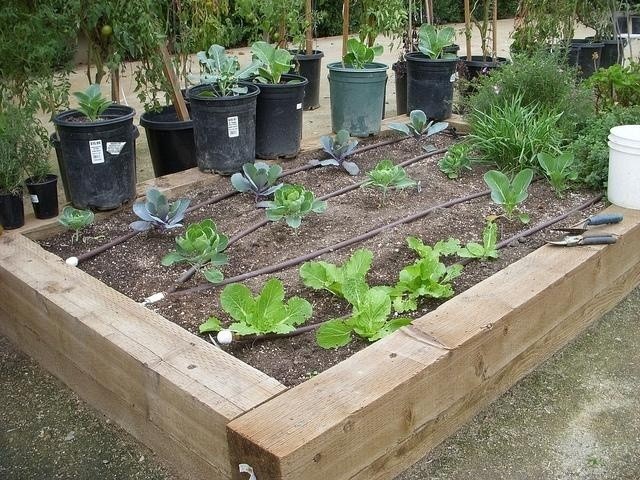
[607,123,640,211]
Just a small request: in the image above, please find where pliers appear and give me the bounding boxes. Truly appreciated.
[545,234,617,247]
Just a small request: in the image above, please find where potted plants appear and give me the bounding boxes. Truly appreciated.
[238,38,310,160]
[2,1,143,212]
[108,2,208,177]
[2,105,63,221]
[509,1,629,80]
[390,0,512,122]
[235,0,326,111]
[0,128,30,233]
[326,0,408,143]
[185,43,261,178]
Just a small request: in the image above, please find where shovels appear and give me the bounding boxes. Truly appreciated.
[551,213,623,235]
